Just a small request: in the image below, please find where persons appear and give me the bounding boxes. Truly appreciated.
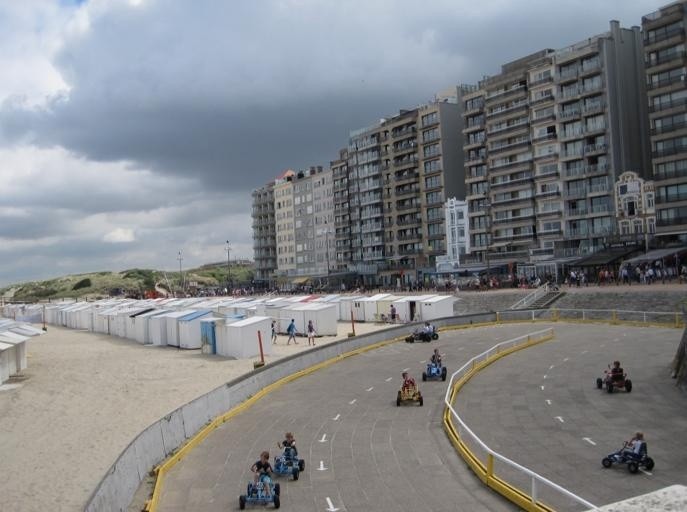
[271,320,277,345]
[390,304,397,324]
[426,323,433,333]
[286,319,299,345]
[607,361,624,381]
[613,431,644,462]
[248,451,273,497]
[186,258,685,298]
[430,348,441,367]
[422,322,428,332]
[401,372,416,393]
[277,431,297,460]
[307,320,316,346]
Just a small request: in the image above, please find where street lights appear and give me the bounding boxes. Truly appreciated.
[176,250,183,283]
[478,189,493,282]
[224,239,231,288]
[321,228,333,276]
